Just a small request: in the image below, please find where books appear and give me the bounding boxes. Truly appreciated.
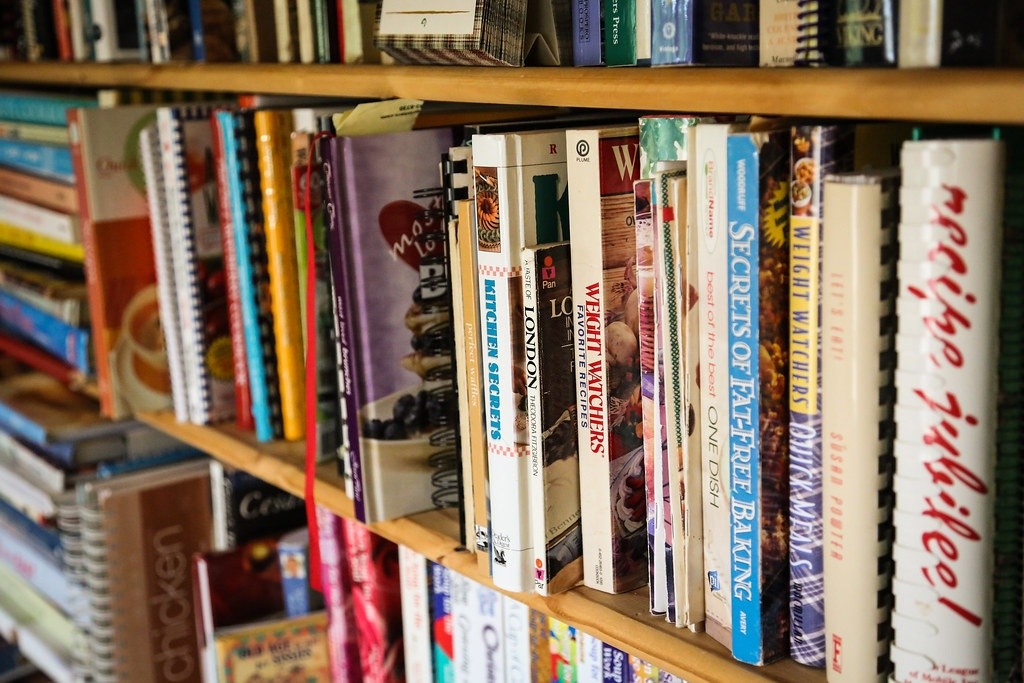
[780,119,869,668]
[819,165,912,682]
[887,127,1008,683]
[0,0,1023,84]
[0,90,789,683]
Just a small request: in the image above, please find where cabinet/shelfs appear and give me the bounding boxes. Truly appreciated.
[1,62,1024,683]
